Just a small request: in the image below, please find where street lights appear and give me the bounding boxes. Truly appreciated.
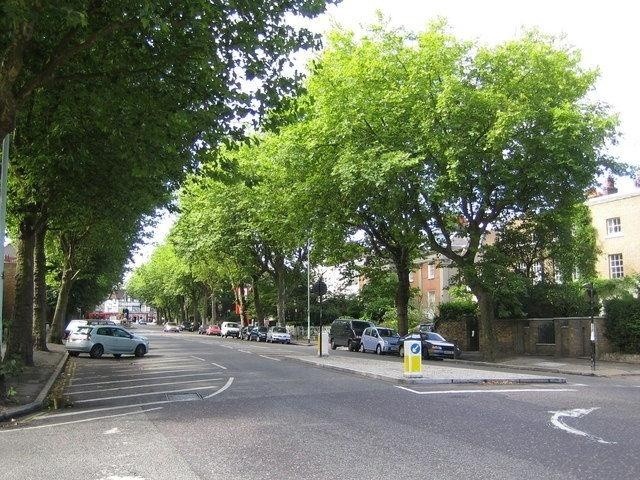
[307,236,316,344]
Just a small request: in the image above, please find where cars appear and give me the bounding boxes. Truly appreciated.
[62,319,150,359]
[360,319,459,361]
[163,321,291,344]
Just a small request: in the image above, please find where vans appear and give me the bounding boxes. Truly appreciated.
[328,319,371,352]
